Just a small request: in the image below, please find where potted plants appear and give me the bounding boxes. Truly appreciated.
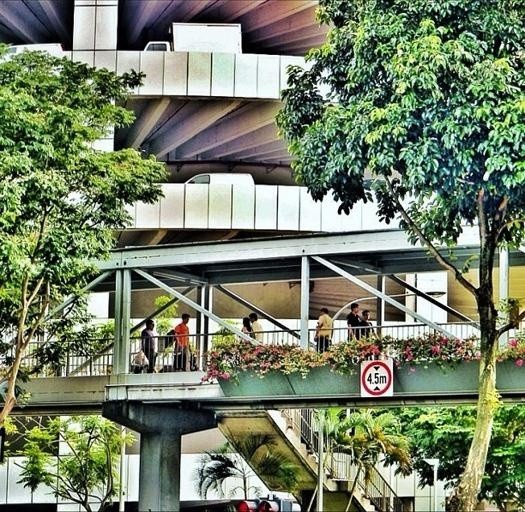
[152,294,179,353]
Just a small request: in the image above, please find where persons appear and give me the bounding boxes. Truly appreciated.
[249,313,264,343]
[361,310,379,339]
[314,307,334,352]
[346,303,365,342]
[173,313,196,370]
[141,318,158,372]
[239,317,256,346]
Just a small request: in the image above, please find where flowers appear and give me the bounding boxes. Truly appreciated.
[198,340,325,385]
[495,322,525,366]
[326,337,479,373]
